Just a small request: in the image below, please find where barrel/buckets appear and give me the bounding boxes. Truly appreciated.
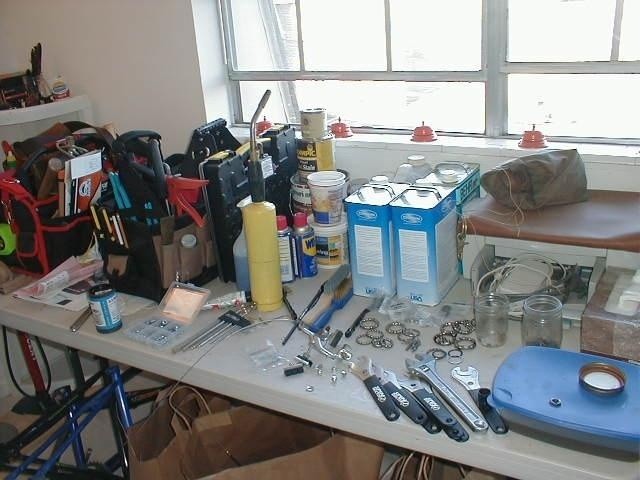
[344,156,480,306]
[288,170,349,221]
[308,212,349,270]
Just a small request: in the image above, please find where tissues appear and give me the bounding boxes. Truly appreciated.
[580,266,640,362]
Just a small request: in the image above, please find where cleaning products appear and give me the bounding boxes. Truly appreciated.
[233,194,253,298]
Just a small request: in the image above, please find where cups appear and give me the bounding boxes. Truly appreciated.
[305,169,346,228]
[520,291,564,353]
[470,290,511,348]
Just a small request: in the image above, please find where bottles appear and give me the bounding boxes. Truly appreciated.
[288,211,319,280]
[273,214,296,283]
[6,150,16,170]
[85,283,124,334]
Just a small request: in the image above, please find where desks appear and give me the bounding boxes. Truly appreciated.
[0,268,640,479]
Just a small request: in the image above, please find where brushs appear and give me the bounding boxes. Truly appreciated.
[301,263,353,332]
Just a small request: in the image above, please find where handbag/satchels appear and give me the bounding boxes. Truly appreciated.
[90,131,220,304]
[180,401,383,479]
[0,120,151,280]
[125,384,232,480]
